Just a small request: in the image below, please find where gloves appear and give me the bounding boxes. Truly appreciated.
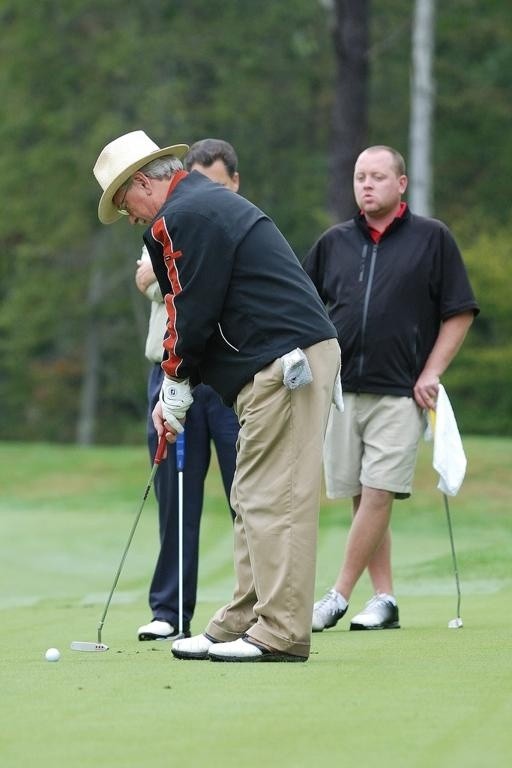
[158,376,196,435]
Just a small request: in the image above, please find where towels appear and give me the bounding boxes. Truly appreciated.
[433,382,468,497]
[279,346,314,391]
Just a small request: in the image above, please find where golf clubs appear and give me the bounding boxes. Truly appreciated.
[71,419,171,652]
[157,423,187,641]
[427,404,463,629]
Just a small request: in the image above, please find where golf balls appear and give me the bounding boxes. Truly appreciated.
[47,648,59,661]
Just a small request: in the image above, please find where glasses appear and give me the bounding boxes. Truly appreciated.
[116,185,131,216]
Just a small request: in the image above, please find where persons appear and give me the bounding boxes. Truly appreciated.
[303,143,478,633]
[89,125,346,663]
[134,138,241,641]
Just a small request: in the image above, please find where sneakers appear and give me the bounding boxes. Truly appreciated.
[207,632,309,664]
[349,591,401,632]
[136,616,192,643]
[170,630,227,661]
[312,587,350,633]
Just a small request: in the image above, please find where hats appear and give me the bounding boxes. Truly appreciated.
[89,128,190,227]
